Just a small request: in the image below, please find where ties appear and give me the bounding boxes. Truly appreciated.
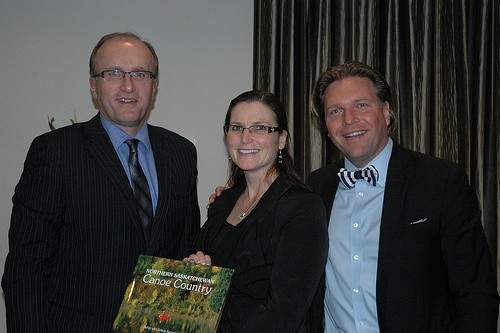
[123,138,155,247]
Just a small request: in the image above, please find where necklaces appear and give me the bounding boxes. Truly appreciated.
[239,175,279,221]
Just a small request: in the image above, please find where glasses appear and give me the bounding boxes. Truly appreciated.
[93,71,156,81]
[224,122,280,138]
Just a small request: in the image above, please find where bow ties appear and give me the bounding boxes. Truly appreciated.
[337,165,379,190]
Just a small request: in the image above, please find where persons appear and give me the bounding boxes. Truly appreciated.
[1,32,201,332]
[206,61,500,333]
[183,90,329,332]
